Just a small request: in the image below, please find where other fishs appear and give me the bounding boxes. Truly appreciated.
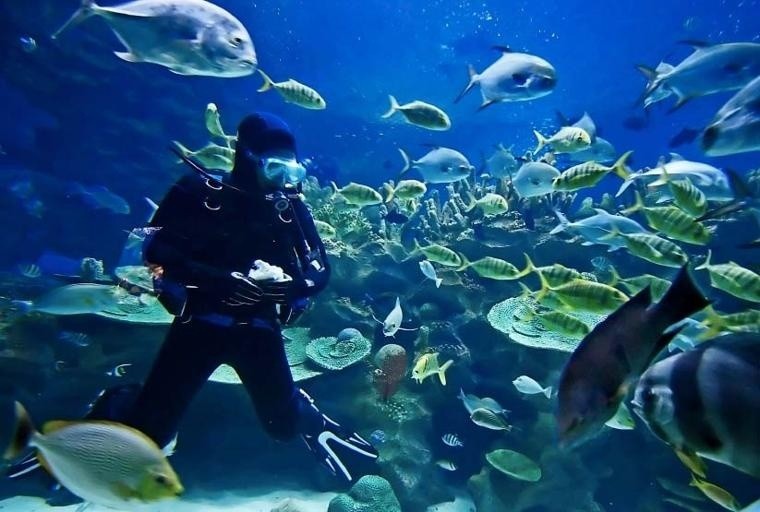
[0,112,759,512]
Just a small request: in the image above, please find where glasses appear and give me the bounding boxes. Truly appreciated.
[243,148,306,185]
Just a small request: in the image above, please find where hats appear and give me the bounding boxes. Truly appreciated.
[234,112,297,186]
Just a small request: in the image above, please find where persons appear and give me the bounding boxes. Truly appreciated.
[9,111,380,506]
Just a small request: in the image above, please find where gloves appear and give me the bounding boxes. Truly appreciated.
[214,269,264,307]
[254,269,293,304]
